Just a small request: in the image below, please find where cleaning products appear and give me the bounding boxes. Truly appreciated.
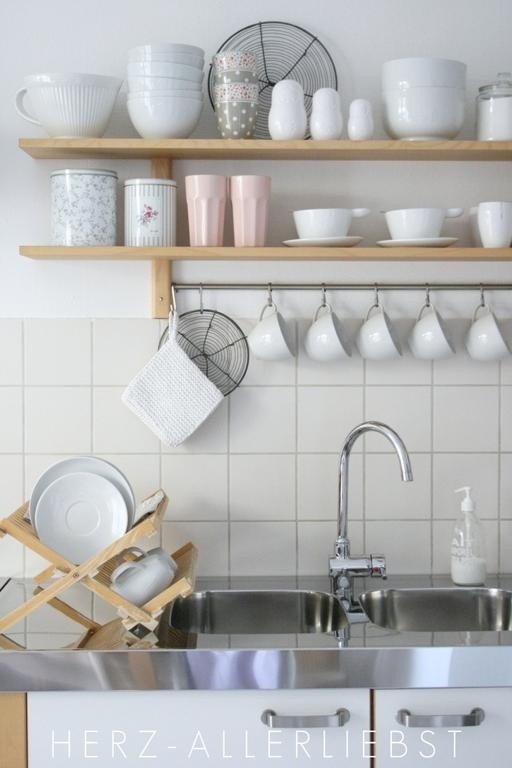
[451,485,490,583]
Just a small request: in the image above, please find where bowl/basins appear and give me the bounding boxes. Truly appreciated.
[124,44,205,139]
[382,89,467,141]
[383,56,467,88]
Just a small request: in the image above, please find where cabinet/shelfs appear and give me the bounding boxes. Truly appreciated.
[26,686,512,767]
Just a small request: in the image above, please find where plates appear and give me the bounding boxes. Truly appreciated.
[34,471,130,566]
[376,236,458,248]
[29,456,137,532]
[283,236,365,246]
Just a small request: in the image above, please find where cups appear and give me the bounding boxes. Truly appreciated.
[292,208,371,237]
[355,303,403,360]
[12,72,123,137]
[477,202,511,247]
[118,547,179,572]
[213,82,260,139]
[464,303,511,363]
[230,174,270,246]
[212,51,257,83]
[469,206,483,249]
[186,173,227,247]
[383,207,464,239]
[247,304,296,358]
[407,304,456,360]
[109,555,175,606]
[303,303,352,362]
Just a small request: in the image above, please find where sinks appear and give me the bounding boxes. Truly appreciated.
[169,574,350,650]
[328,417,413,595]
[358,574,512,647]
